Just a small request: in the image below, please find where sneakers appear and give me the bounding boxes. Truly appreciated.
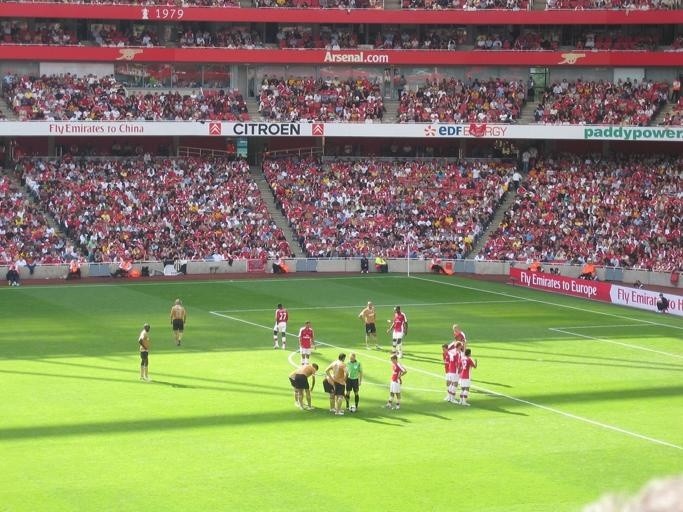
[445,396,469,405]
[295,402,400,415]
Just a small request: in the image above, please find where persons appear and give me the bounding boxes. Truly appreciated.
[0,0,683,290]
[656,293,670,314]
[451,323,466,344]
[272,303,287,350]
[386,306,408,359]
[288,341,476,415]
[297,322,314,366]
[138,323,150,381]
[357,301,380,350]
[169,297,186,345]
